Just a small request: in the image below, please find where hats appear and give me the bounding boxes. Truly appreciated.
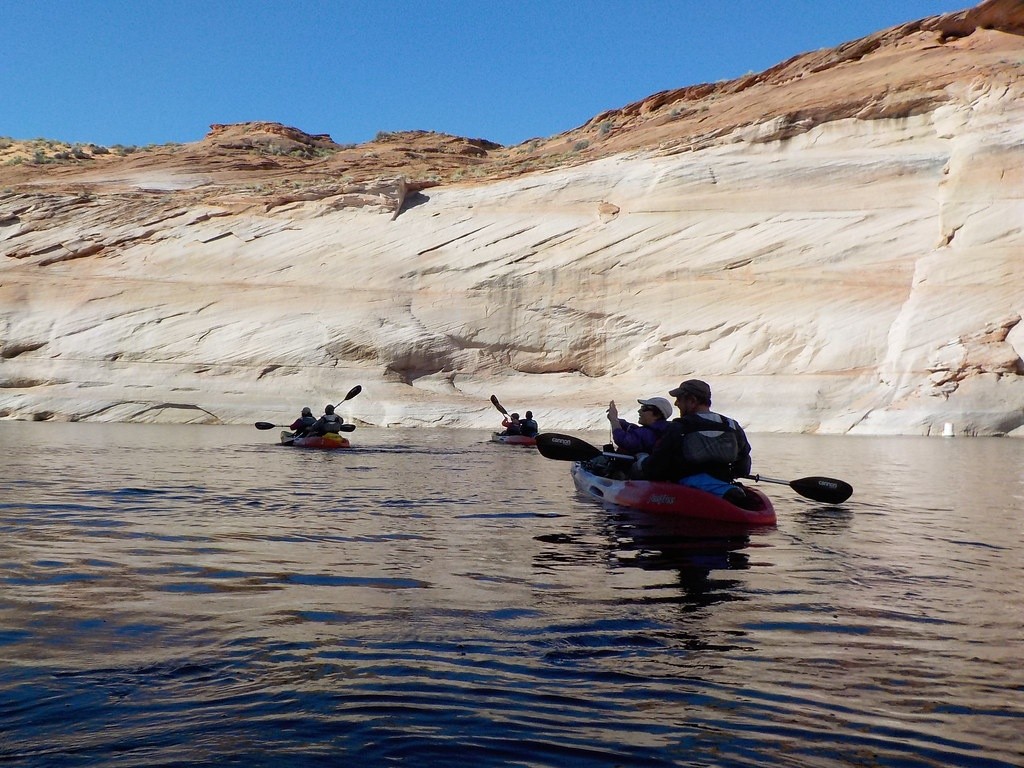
[638,398,672,419]
[669,379,711,398]
[511,413,519,419]
[301,407,311,415]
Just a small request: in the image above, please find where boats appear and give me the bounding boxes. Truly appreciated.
[281,431,349,448]
[491,431,536,445]
[570,460,776,526]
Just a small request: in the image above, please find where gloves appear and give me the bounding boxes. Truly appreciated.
[634,453,649,461]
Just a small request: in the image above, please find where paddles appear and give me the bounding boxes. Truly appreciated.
[254,421,356,432]
[534,433,854,503]
[283,385,362,446]
[489,395,512,420]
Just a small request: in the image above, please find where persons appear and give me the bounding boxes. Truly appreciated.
[289,404,344,439]
[496,411,539,436]
[604,379,752,483]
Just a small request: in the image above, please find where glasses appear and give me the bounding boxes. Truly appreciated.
[641,405,655,412]
[511,418,513,421]
